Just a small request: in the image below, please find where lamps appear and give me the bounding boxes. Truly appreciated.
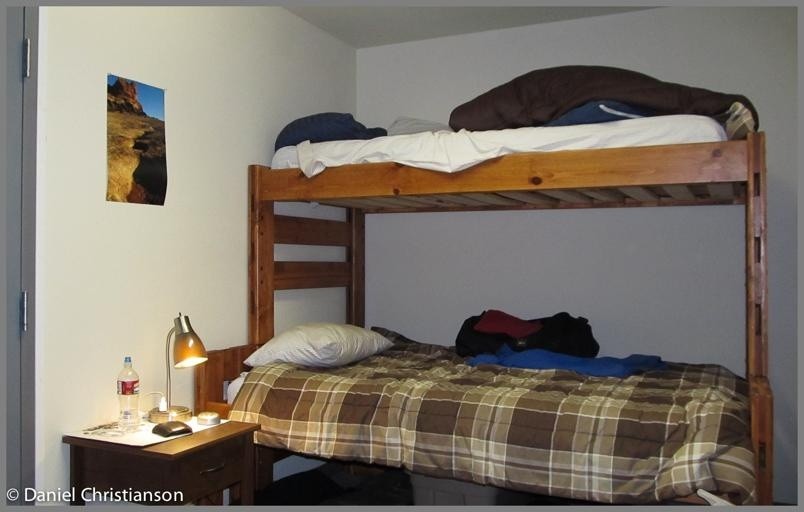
[149,312,208,423]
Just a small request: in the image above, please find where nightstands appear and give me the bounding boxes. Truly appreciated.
[62,416,261,505]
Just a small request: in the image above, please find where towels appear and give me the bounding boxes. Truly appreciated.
[464,343,672,378]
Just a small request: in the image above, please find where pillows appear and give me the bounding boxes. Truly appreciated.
[243,322,395,367]
[275,112,387,152]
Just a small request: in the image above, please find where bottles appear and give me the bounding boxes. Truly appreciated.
[113,357,141,431]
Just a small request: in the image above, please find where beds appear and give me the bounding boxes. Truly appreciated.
[194,132,773,505]
